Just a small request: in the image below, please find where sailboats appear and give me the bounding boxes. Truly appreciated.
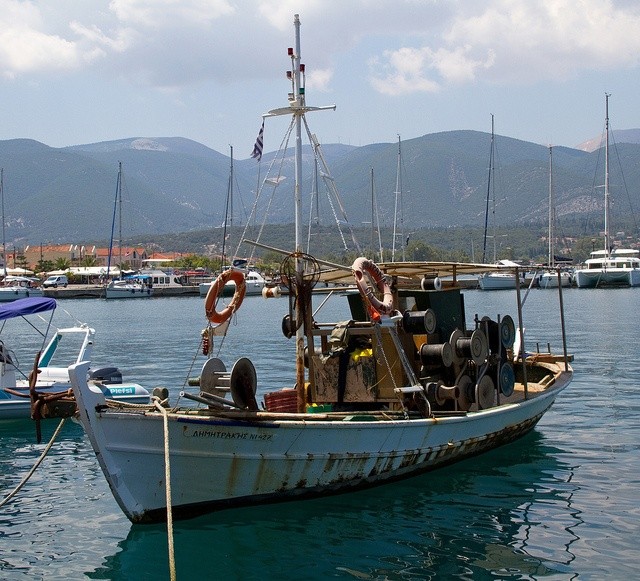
[0,166,45,301]
[479,111,520,290]
[200,144,268,297]
[105,160,151,298]
[278,157,356,295]
[67,13,576,525]
[538,146,572,288]
[575,90,640,288]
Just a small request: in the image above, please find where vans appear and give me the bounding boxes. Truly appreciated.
[43,275,68,288]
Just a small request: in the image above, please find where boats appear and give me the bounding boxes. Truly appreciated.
[0,296,151,419]
[105,276,155,299]
